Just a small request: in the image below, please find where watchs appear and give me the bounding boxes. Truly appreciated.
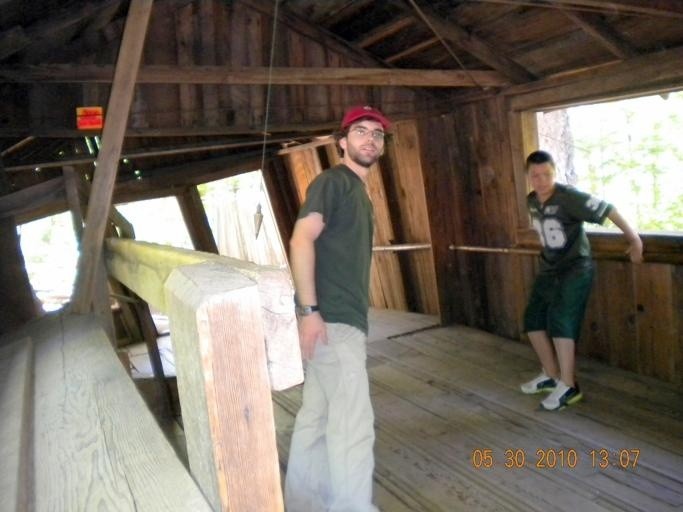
[298,304,320,317]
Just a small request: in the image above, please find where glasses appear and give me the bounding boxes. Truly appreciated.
[349,129,385,138]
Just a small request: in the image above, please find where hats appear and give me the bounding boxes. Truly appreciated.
[340,106,389,131]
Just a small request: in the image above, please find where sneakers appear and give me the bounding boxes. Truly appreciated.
[539,380,582,412]
[520,375,559,394]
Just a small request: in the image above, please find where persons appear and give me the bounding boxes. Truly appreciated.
[283,107,392,512]
[521,149,645,410]
